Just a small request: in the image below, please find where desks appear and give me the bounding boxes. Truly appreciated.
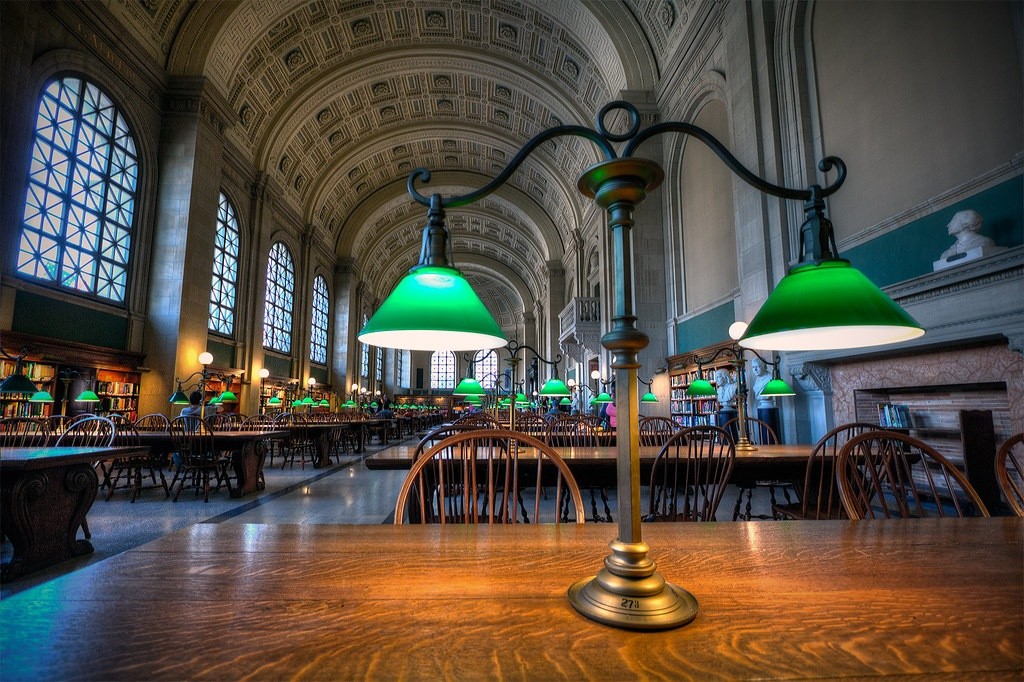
[363,416,1024,489]
[0,513,1024,682]
[0,416,446,580]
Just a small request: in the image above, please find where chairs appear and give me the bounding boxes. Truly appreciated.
[0,410,1024,526]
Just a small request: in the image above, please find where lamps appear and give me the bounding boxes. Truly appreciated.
[356,99,925,630]
[0,346,331,433]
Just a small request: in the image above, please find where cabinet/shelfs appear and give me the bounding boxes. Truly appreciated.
[0,356,59,433]
[205,365,245,425]
[664,339,745,443]
[89,368,142,427]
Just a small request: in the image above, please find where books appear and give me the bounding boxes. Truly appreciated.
[877,404,912,428]
[729,371,742,383]
[95,382,138,423]
[671,370,719,442]
[0,359,54,432]
[261,388,295,414]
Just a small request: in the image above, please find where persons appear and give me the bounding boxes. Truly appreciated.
[544,400,564,415]
[752,356,773,400]
[596,381,616,447]
[171,391,218,473]
[363,395,439,445]
[587,389,596,410]
[714,369,739,411]
[469,404,473,413]
[571,393,579,410]
[940,209,996,260]
